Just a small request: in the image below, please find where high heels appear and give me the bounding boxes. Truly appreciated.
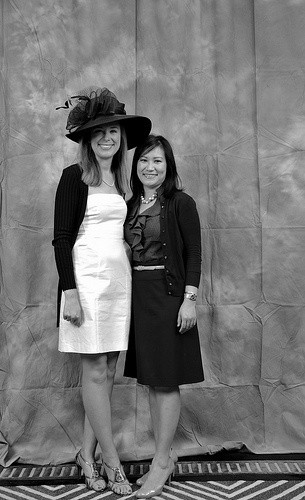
[135,459,175,498]
[75,450,106,492]
[100,454,133,495]
[137,448,178,485]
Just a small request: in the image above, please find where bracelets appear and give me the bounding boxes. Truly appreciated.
[184,291,197,301]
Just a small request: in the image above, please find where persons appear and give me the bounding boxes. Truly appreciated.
[123,134,207,498]
[51,87,152,495]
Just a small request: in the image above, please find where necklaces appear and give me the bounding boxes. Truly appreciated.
[141,194,158,204]
[102,178,114,187]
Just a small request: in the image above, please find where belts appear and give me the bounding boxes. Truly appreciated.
[134,265,165,271]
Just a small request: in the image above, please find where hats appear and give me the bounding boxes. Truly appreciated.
[56,88,152,150]
